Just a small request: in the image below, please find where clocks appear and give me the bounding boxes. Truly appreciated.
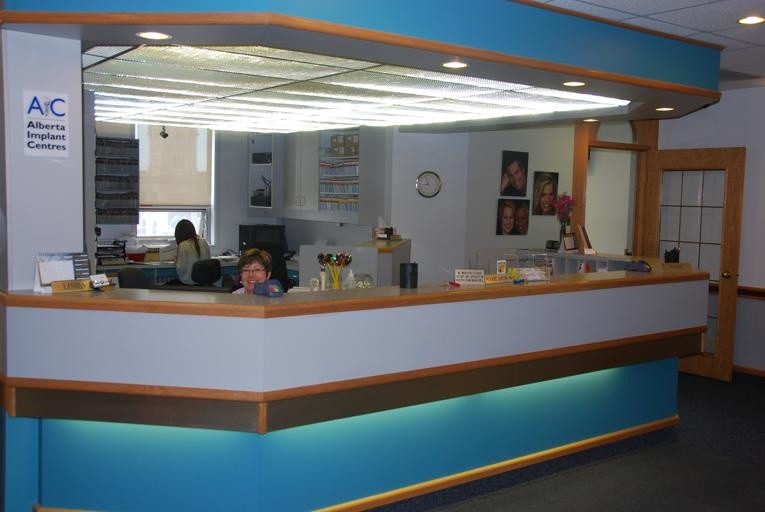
[415,171,442,198]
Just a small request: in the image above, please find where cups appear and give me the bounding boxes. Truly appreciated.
[400,262,418,289]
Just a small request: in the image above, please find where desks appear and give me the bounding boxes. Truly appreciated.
[95,253,300,287]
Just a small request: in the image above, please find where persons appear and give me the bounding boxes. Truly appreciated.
[532,172,557,215]
[512,200,529,235]
[230,247,273,296]
[250,229,298,293]
[498,198,516,235]
[163,219,213,287]
[499,155,527,197]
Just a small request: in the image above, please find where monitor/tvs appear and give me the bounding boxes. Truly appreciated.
[149,284,233,293]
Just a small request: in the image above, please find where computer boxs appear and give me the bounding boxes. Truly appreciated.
[238,224,286,259]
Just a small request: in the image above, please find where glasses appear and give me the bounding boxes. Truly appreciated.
[239,267,266,276]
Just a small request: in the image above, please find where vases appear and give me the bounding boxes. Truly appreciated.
[559,227,566,244]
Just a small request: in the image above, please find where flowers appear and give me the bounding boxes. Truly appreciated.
[545,192,576,226]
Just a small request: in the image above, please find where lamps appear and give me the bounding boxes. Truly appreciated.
[160,125,168,139]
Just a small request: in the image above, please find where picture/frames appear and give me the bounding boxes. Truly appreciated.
[248,133,275,210]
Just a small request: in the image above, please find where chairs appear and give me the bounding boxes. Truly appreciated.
[189,258,223,291]
[117,266,154,288]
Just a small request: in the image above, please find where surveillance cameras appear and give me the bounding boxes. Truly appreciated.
[160,131,168,139]
